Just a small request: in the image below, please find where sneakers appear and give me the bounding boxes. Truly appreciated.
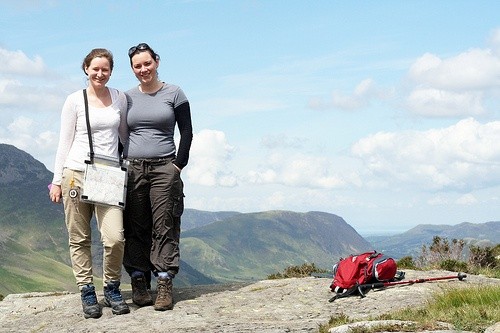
[103,279,130,315]
[131,271,154,306]
[154,272,174,311]
[79,283,101,319]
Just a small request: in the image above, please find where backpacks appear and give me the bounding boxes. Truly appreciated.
[330,250,397,295]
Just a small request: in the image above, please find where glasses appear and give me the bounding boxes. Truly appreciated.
[128,43,147,56]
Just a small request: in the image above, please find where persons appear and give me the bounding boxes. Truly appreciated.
[117,44,192,311]
[50,48,130,318]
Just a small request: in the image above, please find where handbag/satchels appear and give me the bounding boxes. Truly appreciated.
[80,152,130,209]
